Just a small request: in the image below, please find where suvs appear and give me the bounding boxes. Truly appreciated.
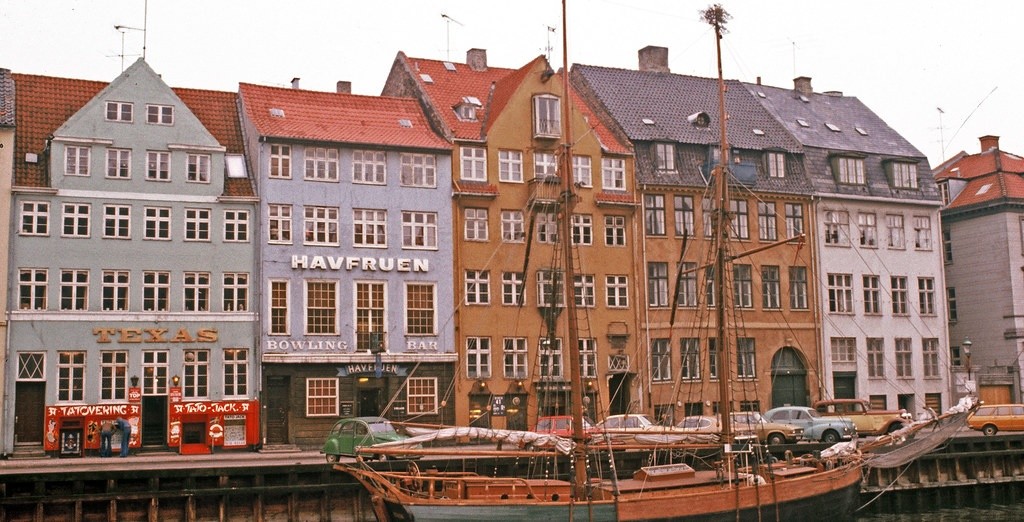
[809,399,914,437]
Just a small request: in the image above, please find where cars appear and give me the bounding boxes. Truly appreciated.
[674,414,739,443]
[761,405,858,444]
[319,417,426,460]
[709,411,805,445]
[594,414,685,443]
[519,415,609,452]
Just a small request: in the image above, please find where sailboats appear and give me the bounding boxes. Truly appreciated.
[331,0,981,522]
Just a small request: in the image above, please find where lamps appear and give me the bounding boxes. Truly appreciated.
[515,377,525,391]
[172,374,181,386]
[478,377,487,391]
[587,376,595,390]
[131,374,139,387]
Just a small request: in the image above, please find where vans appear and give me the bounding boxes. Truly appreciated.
[967,403,1024,435]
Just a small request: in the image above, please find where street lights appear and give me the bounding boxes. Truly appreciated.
[962,336,973,395]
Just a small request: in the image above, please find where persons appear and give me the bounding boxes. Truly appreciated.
[116,418,131,457]
[99,422,117,457]
